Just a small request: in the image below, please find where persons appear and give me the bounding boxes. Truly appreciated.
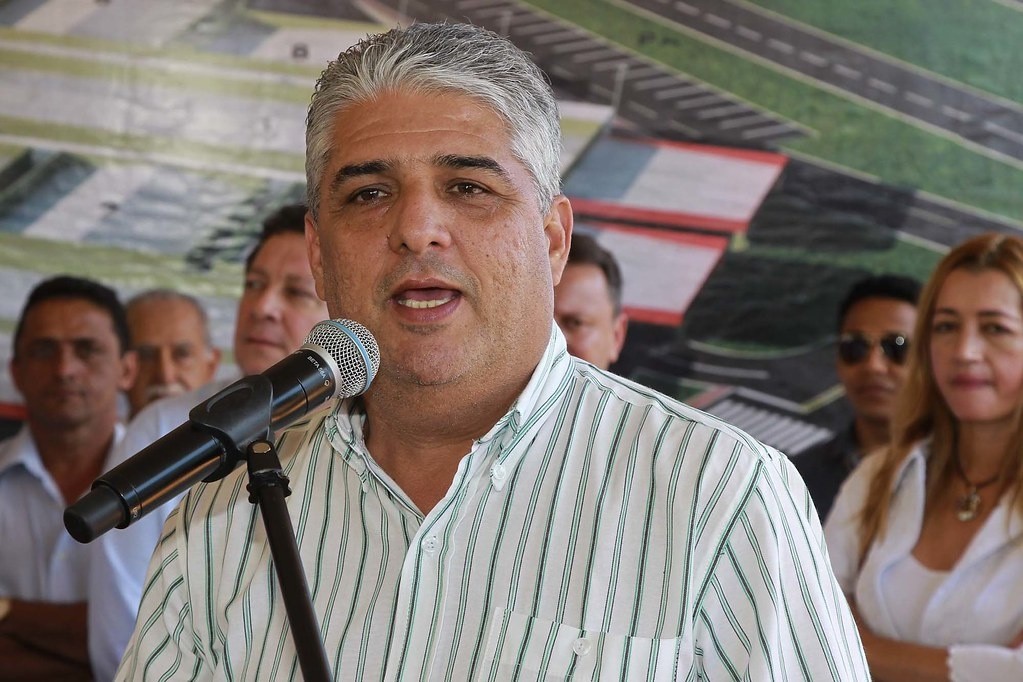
[554,233,629,371]
[0,273,140,682]
[124,288,223,422]
[821,233,1023,682]
[789,275,924,525]
[112,5,873,682]
[87,203,330,682]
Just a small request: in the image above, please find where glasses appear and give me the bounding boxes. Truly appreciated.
[837,333,915,364]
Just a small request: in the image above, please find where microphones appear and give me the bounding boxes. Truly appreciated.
[63,318,381,544]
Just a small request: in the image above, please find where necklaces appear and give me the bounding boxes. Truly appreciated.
[954,448,999,522]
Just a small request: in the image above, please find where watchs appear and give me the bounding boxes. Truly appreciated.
[0,598,12,623]
